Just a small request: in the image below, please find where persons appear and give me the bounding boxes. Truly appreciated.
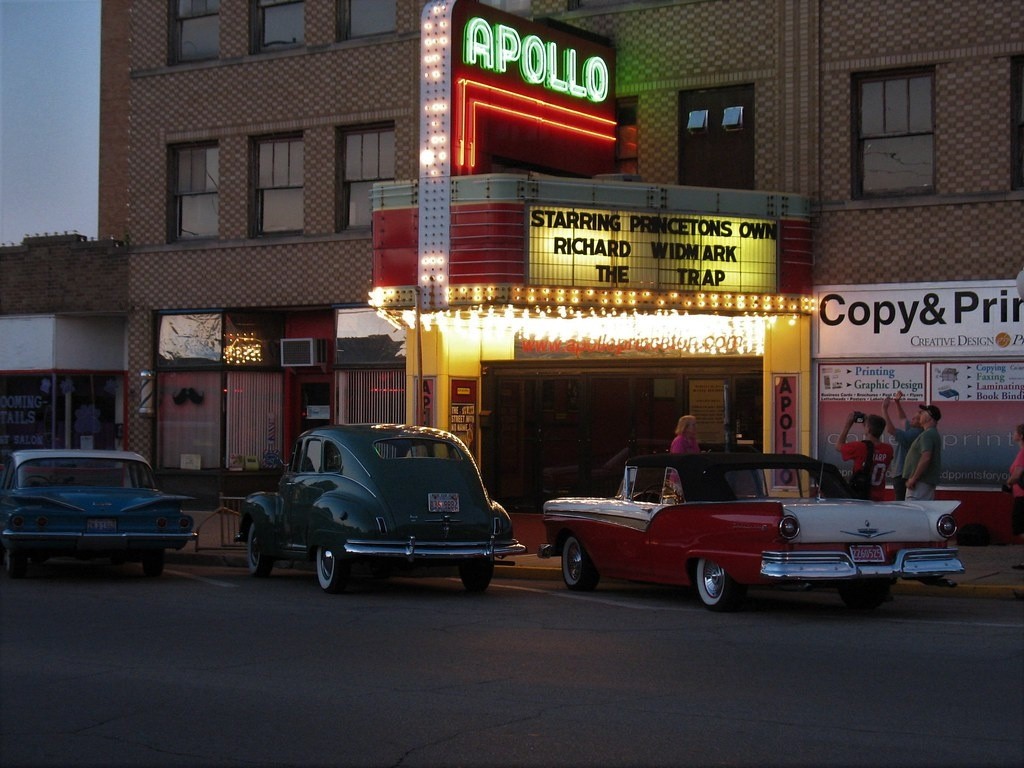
[902,404,941,501]
[882,391,923,501]
[1006,423,1024,536]
[835,411,894,501]
[670,415,701,454]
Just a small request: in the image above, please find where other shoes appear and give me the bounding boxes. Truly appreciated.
[1012,563,1024,570]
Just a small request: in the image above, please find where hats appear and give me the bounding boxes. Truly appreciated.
[918,404,941,421]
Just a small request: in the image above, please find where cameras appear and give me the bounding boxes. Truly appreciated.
[854,411,866,423]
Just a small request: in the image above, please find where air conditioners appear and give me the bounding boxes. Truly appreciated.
[280,337,326,369]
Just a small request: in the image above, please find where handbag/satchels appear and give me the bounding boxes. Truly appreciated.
[849,440,873,499]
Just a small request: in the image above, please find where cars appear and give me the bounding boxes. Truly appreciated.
[537,450,967,615]
[0,447,198,578]
[233,423,527,595]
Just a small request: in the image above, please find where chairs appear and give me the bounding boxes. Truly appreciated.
[719,465,774,501]
